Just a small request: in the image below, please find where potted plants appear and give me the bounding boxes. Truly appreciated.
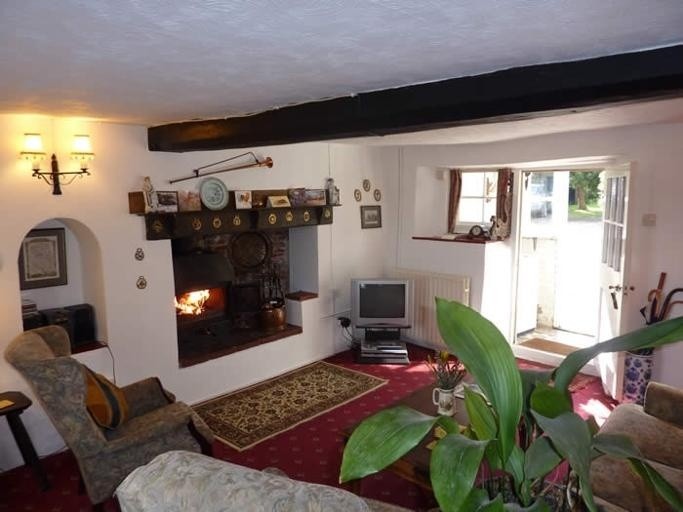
[425,349,467,418]
[337,293,683,512]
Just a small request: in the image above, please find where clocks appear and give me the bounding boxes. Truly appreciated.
[468,224,484,239]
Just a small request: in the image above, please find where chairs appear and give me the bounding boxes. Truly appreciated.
[111,449,415,512]
[585,381,683,512]
[4,324,212,510]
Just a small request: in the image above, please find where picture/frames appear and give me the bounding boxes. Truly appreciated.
[155,190,179,213]
[360,205,382,229]
[19,226,69,290]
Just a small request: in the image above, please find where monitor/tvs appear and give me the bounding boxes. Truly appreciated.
[351,277,414,327]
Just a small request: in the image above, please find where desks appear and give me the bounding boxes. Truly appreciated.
[0,390,53,491]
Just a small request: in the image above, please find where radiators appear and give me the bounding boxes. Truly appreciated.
[387,267,471,355]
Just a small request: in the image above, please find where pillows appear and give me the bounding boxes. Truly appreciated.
[82,361,130,431]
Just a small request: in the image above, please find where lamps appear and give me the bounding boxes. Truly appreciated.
[19,131,95,195]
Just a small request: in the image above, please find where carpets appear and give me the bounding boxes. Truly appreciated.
[189,360,389,452]
[541,365,594,393]
[518,336,583,359]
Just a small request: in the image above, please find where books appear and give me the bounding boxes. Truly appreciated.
[20,297,40,321]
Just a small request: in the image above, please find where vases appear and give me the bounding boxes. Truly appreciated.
[621,353,653,407]
